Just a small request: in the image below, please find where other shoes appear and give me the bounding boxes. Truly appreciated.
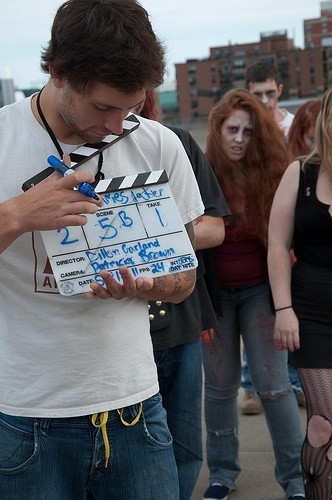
[203,482,231,500]
[240,389,261,414]
[289,493,305,500]
[295,394,306,405]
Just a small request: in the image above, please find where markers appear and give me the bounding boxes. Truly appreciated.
[47,154,100,201]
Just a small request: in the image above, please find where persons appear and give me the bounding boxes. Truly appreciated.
[0,1,206,500]
[201,86,313,499]
[268,87,332,500]
[240,61,308,415]
[139,87,232,500]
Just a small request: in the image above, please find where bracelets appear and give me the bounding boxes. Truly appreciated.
[275,306,292,311]
